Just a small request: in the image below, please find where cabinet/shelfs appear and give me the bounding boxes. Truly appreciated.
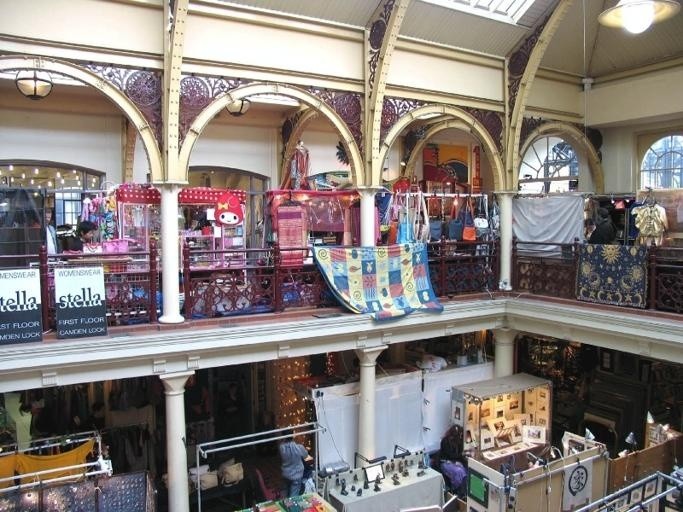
[0,185,48,267]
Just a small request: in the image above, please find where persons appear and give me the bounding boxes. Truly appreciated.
[78,401,105,431]
[589,208,610,243]
[439,425,467,500]
[13,207,97,255]
[278,429,308,498]
[604,204,617,241]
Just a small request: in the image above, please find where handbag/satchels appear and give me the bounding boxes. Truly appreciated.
[387,206,430,243]
[430,221,442,239]
[103,242,129,255]
[218,458,244,483]
[63,250,83,258]
[189,465,219,491]
[446,211,489,241]
[83,244,103,254]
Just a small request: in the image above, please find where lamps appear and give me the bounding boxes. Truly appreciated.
[226,98,251,117]
[596,1,681,36]
[16,68,53,101]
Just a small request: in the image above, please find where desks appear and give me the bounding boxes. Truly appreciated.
[154,478,252,511]
[328,464,445,511]
[239,491,335,512]
